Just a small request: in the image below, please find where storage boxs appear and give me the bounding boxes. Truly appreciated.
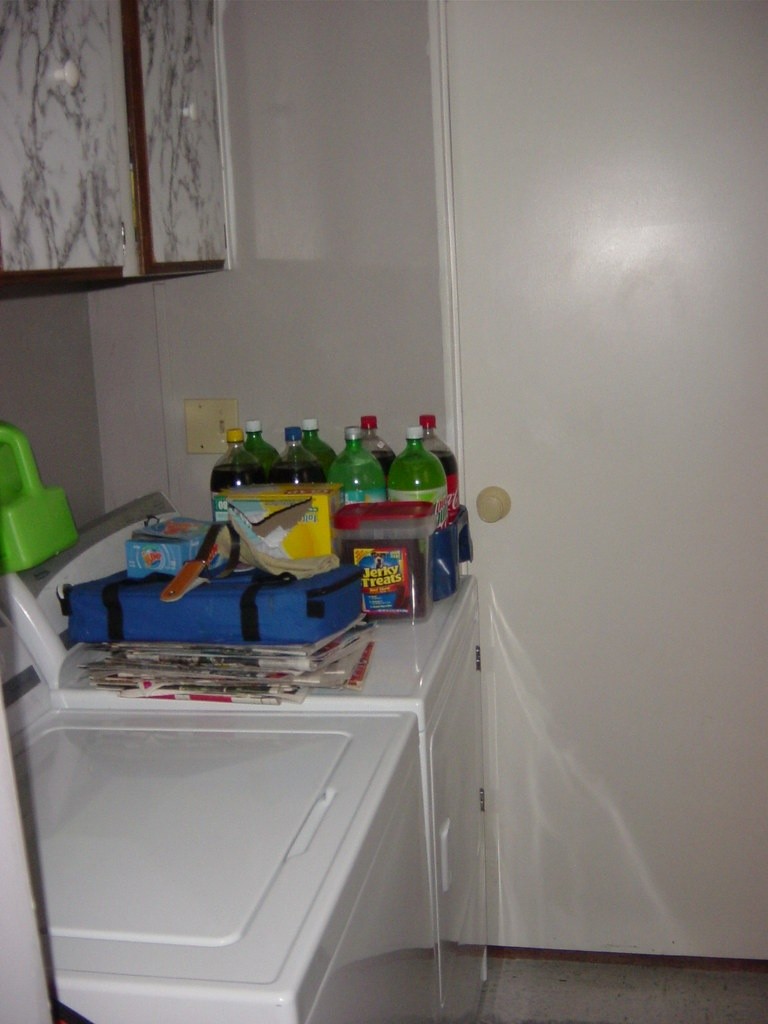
[126,517,212,579]
[57,559,365,648]
[210,485,344,559]
[332,501,436,624]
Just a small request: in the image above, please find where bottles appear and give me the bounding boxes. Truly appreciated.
[244,420,280,485]
[420,415,460,526]
[209,429,258,522]
[301,419,337,484]
[361,416,396,502]
[327,426,386,506]
[388,425,448,532]
[269,426,326,484]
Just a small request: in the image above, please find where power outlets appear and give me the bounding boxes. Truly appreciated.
[184,399,240,455]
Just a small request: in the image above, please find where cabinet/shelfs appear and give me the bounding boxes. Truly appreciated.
[0,0,239,303]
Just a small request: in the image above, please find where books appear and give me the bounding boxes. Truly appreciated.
[77,612,379,707]
